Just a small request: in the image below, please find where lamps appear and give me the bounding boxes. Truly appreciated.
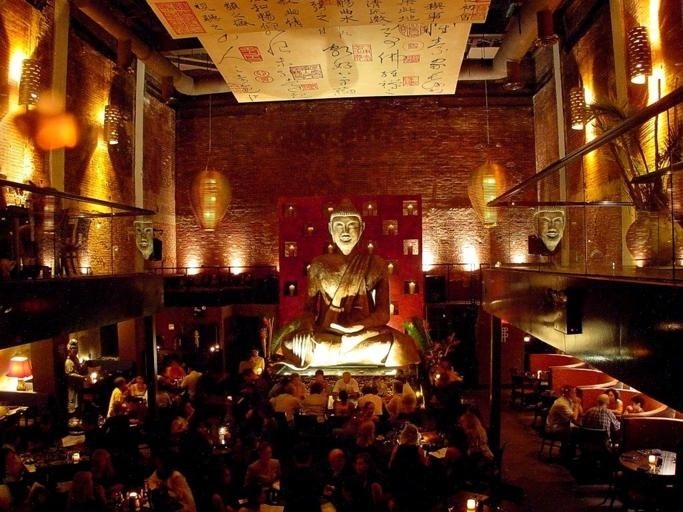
[18,58,42,106]
[467,23,513,228]
[570,87,585,130]
[187,53,233,231]
[626,25,652,84]
[104,105,121,145]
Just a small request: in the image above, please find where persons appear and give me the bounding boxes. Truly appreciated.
[623,396,645,415]
[268,206,421,369]
[572,394,619,446]
[501,204,632,356]
[604,387,622,415]
[543,386,581,458]
[132,217,156,259]
[0,326,494,512]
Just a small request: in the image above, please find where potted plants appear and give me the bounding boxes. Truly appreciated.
[582,91,683,268]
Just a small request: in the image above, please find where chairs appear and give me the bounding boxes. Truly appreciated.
[0,175,90,279]
[0,354,683,512]
